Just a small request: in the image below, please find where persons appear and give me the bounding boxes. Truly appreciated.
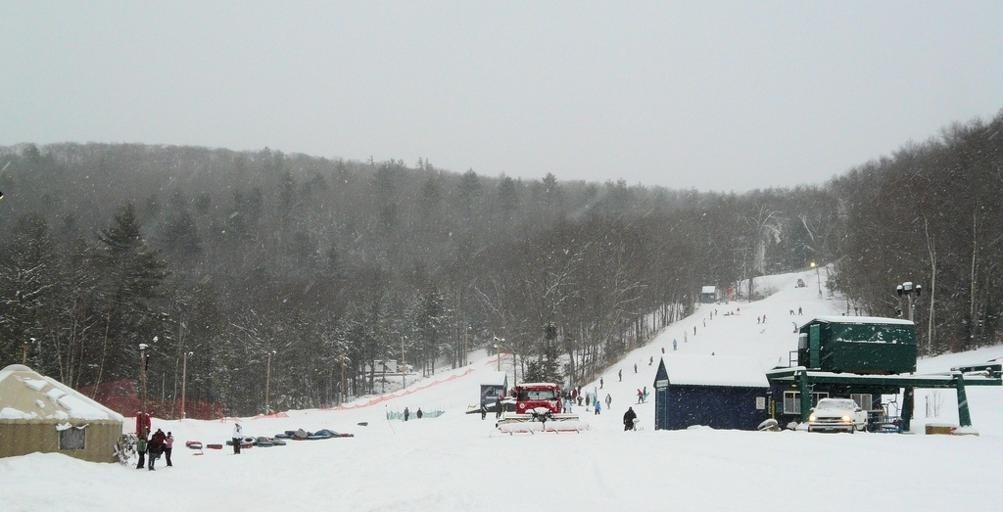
[757,315,760,324]
[482,399,486,420]
[153,428,166,460]
[649,355,654,365]
[635,388,643,403]
[135,434,146,469]
[598,376,603,389]
[642,386,647,402]
[561,384,596,413]
[788,309,795,316]
[703,318,706,327]
[604,392,611,409]
[494,398,502,418]
[660,346,664,354]
[796,306,803,315]
[714,307,717,316]
[709,310,712,320]
[693,326,696,335]
[617,368,622,382]
[761,313,767,324]
[231,422,242,454]
[672,337,677,351]
[416,406,422,418]
[164,431,173,466]
[634,363,637,373]
[404,406,408,421]
[621,405,637,431]
[146,434,159,470]
[683,330,687,343]
[594,400,601,414]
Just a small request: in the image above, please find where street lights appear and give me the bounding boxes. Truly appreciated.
[810,262,822,296]
[265,351,276,416]
[181,351,193,420]
[139,335,159,441]
[897,281,922,321]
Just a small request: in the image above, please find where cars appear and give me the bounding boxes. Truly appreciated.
[808,398,869,434]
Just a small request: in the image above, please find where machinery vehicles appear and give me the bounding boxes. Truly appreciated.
[495,382,584,436]
